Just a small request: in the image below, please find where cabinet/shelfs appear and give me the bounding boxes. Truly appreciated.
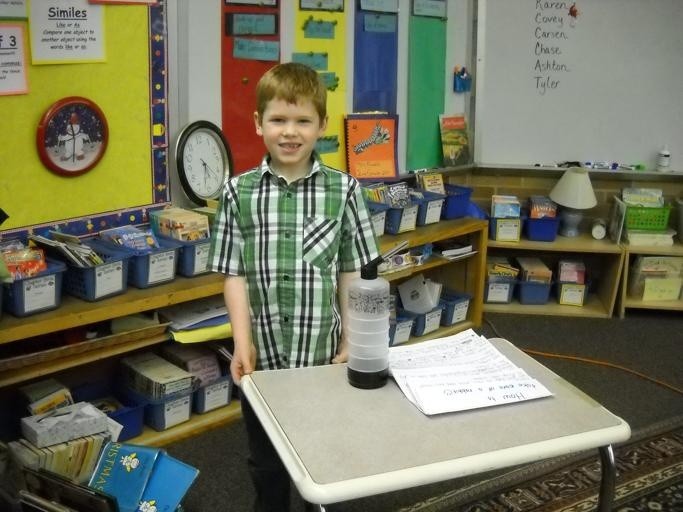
[618,236,683,321]
[483,235,624,321]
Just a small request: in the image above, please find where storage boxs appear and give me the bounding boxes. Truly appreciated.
[485,209,591,306]
[628,266,683,306]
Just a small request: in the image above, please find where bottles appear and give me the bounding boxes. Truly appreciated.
[345,263,392,390]
[591,218,607,240]
[656,144,671,173]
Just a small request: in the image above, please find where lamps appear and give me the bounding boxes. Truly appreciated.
[548,166,600,238]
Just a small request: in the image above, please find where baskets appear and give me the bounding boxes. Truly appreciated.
[66,338,249,444]
[484,260,592,307]
[624,201,673,230]
[359,180,474,238]
[1,221,221,319]
[486,201,562,243]
[409,241,432,262]
[387,284,473,347]
[453,67,472,93]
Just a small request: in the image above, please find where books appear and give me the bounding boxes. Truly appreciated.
[102,227,159,251]
[432,249,477,259]
[436,242,473,254]
[1,247,47,283]
[396,274,433,315]
[438,113,471,168]
[618,187,682,303]
[426,279,443,308]
[416,172,445,195]
[363,182,411,208]
[1,342,232,512]
[28,230,103,267]
[150,198,220,243]
[343,110,402,185]
[486,194,585,283]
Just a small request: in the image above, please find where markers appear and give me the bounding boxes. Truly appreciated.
[584,162,635,173]
[534,163,557,169]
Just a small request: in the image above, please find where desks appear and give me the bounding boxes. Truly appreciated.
[234,322,632,510]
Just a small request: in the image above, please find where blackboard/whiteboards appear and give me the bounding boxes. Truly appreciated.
[167,1,473,199]
[475,0,683,181]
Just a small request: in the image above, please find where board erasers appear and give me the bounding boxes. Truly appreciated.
[558,162,579,168]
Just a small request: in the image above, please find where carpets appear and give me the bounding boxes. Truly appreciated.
[400,397,682,512]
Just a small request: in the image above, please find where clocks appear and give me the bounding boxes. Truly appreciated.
[174,119,234,208]
[32,93,111,180]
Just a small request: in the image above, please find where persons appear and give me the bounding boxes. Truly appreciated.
[205,62,382,510]
